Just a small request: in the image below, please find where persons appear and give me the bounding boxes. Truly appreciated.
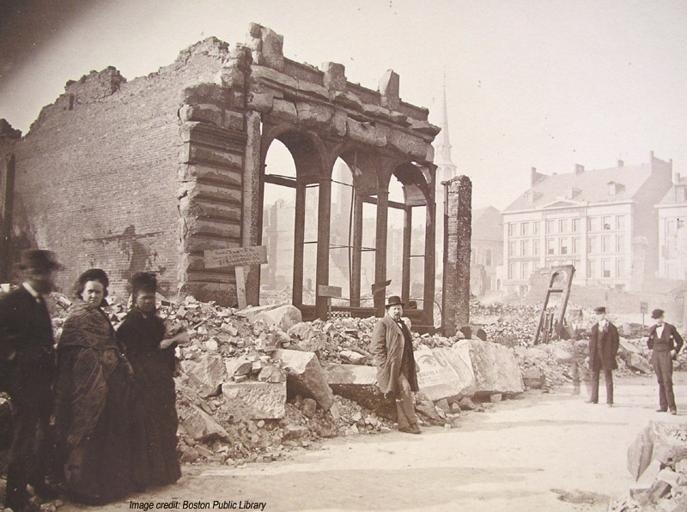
[45,269,135,505]
[646,310,684,415]
[0,250,63,512]
[116,272,185,492]
[586,307,619,407]
[374,297,421,433]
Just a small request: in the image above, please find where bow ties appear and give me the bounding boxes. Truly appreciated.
[656,325,662,328]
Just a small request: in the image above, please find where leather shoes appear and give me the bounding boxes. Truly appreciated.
[399,423,420,433]
[5,484,59,511]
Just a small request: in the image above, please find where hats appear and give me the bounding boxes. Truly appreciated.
[594,306,605,312]
[15,250,59,269]
[651,309,664,319]
[385,296,405,309]
